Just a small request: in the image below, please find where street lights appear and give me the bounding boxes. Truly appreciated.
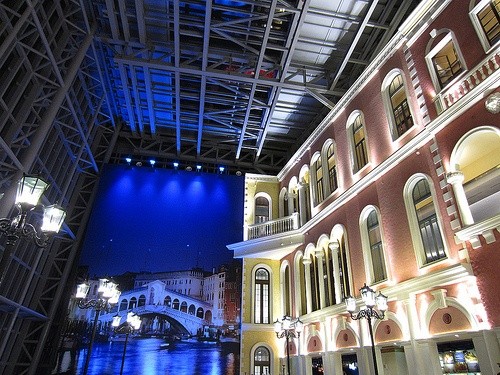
[273,312,304,375]
[75,273,122,375]
[0,170,69,280]
[111,309,142,375]
[341,280,391,375]
